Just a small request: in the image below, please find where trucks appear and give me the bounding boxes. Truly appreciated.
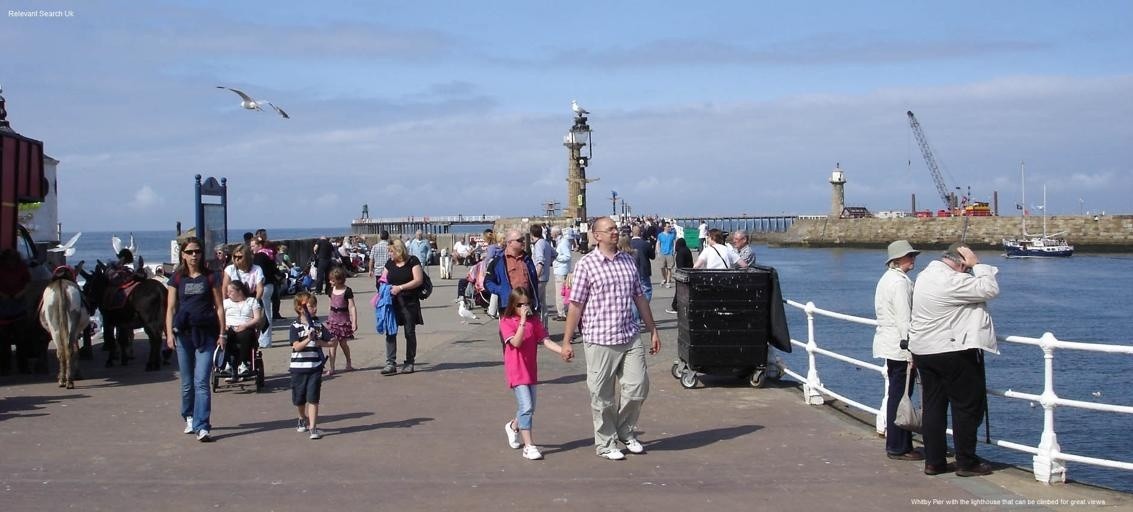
[0,132,53,372]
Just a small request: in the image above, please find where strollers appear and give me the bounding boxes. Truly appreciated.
[286,257,316,295]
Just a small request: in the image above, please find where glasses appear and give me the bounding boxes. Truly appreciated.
[511,235,526,243]
[182,248,201,256]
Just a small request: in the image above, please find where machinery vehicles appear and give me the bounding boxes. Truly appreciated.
[904,108,967,216]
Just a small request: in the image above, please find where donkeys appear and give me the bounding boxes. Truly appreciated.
[37,259,90,392]
[75,262,169,372]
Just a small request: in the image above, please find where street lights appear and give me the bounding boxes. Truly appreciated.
[619,199,631,216]
[565,116,600,253]
[607,190,619,215]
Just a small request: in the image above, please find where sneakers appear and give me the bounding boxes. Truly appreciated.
[400,360,415,374]
[380,363,399,377]
[183,415,196,434]
[219,361,250,382]
[955,461,994,477]
[271,312,287,322]
[659,280,679,314]
[326,367,336,376]
[552,314,568,322]
[195,429,212,443]
[596,448,626,461]
[297,416,308,433]
[520,443,544,460]
[308,426,321,439]
[923,459,950,476]
[883,448,926,462]
[620,437,645,454]
[344,358,354,371]
[504,419,521,450]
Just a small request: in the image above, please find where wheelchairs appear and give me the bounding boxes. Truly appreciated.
[209,322,265,394]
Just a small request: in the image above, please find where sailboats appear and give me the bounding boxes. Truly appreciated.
[1000,158,1075,259]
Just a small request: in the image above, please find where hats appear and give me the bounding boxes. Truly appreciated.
[947,240,972,259]
[881,238,921,266]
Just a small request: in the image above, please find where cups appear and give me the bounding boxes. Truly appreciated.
[522,304,533,320]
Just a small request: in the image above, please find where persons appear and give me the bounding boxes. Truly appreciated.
[873,240,923,461]
[908,241,1000,476]
[0,248,33,374]
[108,249,133,345]
[166,238,225,441]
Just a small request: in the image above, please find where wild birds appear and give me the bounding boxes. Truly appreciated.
[216,85,290,119]
[111,230,137,261]
[46,231,82,257]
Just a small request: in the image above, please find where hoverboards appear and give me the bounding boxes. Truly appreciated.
[669,265,774,389]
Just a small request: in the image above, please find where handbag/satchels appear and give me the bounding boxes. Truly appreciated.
[891,361,927,434]
[414,271,434,301]
